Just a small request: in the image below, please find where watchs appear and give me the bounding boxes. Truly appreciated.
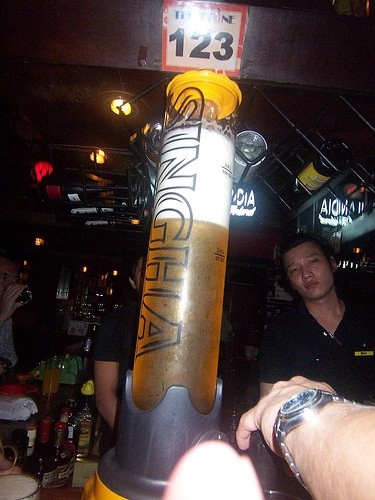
[272,387,364,493]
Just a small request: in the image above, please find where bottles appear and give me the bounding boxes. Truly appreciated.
[5,353,96,489]
[82,324,94,353]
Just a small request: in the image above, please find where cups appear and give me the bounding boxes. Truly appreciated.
[249,430,309,500]
[3,283,32,304]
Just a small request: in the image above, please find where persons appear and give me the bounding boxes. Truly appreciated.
[0,231,375,500]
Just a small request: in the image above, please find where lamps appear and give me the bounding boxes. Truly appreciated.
[90,68,150,121]
